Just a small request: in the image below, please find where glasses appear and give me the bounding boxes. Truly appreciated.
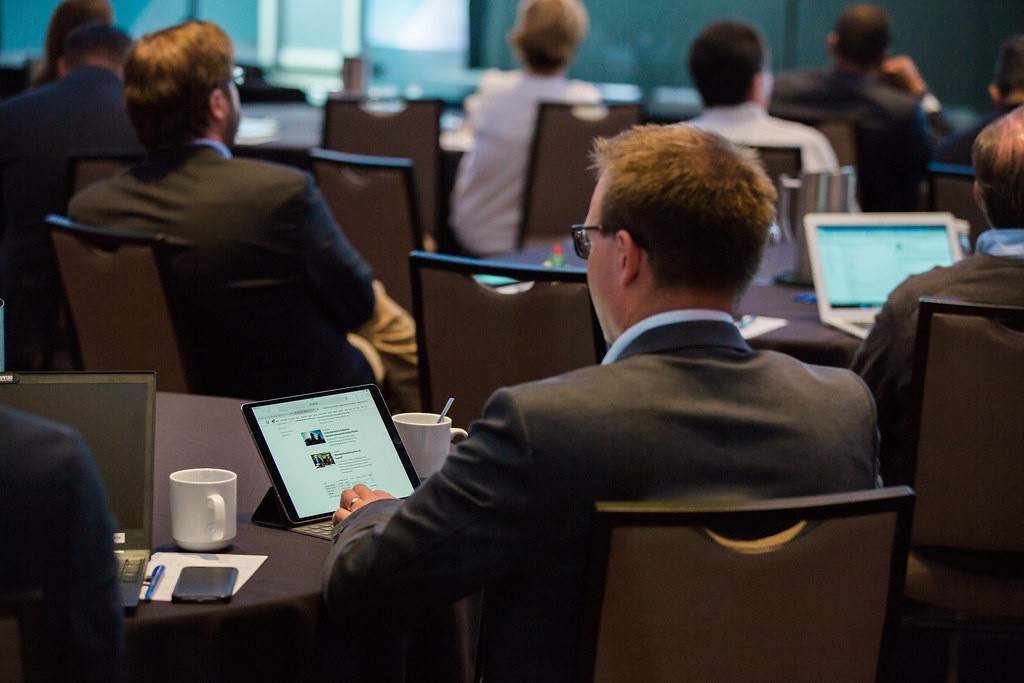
[571,223,618,259]
[221,67,244,86]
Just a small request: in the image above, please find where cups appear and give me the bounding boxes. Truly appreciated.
[168,468,238,551]
[344,58,365,99]
[392,412,469,478]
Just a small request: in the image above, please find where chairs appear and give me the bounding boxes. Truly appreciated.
[41,94,1024,682]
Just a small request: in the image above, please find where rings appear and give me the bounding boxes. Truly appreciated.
[351,498,358,506]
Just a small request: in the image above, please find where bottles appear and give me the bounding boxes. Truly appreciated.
[552,243,565,269]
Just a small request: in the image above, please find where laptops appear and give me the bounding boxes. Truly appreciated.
[802,211,965,341]
[241,383,424,540]
[0,370,156,610]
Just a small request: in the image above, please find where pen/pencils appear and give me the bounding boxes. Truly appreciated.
[145,565,165,601]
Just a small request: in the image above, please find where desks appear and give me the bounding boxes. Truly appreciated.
[105,388,460,683]
[486,231,861,367]
[230,99,464,170]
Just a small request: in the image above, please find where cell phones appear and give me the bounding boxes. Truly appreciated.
[172,566,239,604]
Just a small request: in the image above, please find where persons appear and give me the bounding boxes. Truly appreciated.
[327,127,878,683]
[684,20,843,174]
[66,22,424,405]
[451,1,609,251]
[1,22,139,376]
[37,2,116,83]
[852,104,1024,560]
[0,406,124,683]
[990,35,1023,111]
[771,3,943,121]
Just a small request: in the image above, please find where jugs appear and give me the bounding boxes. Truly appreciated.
[782,168,858,281]
